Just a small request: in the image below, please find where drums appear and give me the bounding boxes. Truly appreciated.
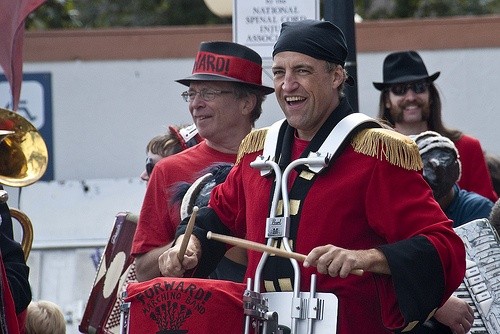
[77,209,139,334]
[118,276,248,334]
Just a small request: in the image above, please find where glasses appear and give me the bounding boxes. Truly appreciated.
[145,160,155,177]
[180,88,236,102]
[387,80,430,95]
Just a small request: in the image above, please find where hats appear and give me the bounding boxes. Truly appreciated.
[372,50,440,92]
[174,41,276,95]
[169,123,204,151]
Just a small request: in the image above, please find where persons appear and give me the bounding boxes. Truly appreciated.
[130,42,275,284]
[0,187,32,312]
[22,300,66,334]
[372,50,498,199]
[159,20,466,334]
[415,126,500,334]
[139,123,203,188]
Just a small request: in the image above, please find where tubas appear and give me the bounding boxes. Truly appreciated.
[0,105,49,270]
